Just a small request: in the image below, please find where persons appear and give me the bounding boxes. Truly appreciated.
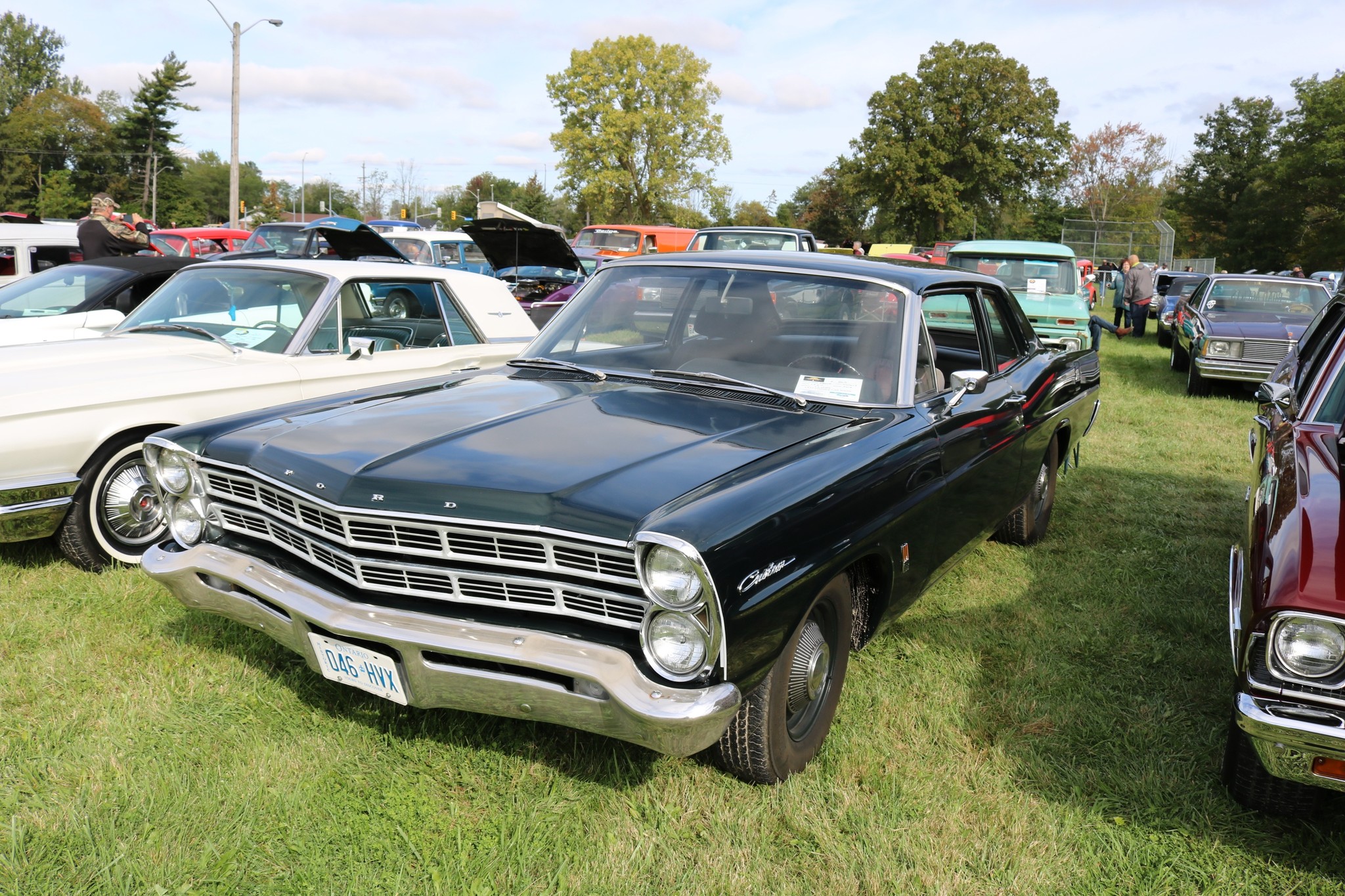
[77,192,150,308]
[917,249,931,259]
[1286,264,1305,301]
[853,241,865,255]
[1088,255,1193,352]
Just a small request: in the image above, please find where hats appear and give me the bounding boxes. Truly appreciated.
[91,192,121,209]
[1291,264,1300,268]
[1163,262,1168,266]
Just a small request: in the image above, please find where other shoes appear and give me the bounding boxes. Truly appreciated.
[1100,296,1105,299]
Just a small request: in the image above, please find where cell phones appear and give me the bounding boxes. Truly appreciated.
[123,214,133,222]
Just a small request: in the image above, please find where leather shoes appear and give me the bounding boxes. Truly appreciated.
[1115,326,1134,340]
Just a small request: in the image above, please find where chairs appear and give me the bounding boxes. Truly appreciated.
[262,327,413,351]
[851,322,945,397]
[672,296,772,366]
[427,330,477,346]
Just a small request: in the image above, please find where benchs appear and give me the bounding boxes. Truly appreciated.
[774,333,1011,388]
